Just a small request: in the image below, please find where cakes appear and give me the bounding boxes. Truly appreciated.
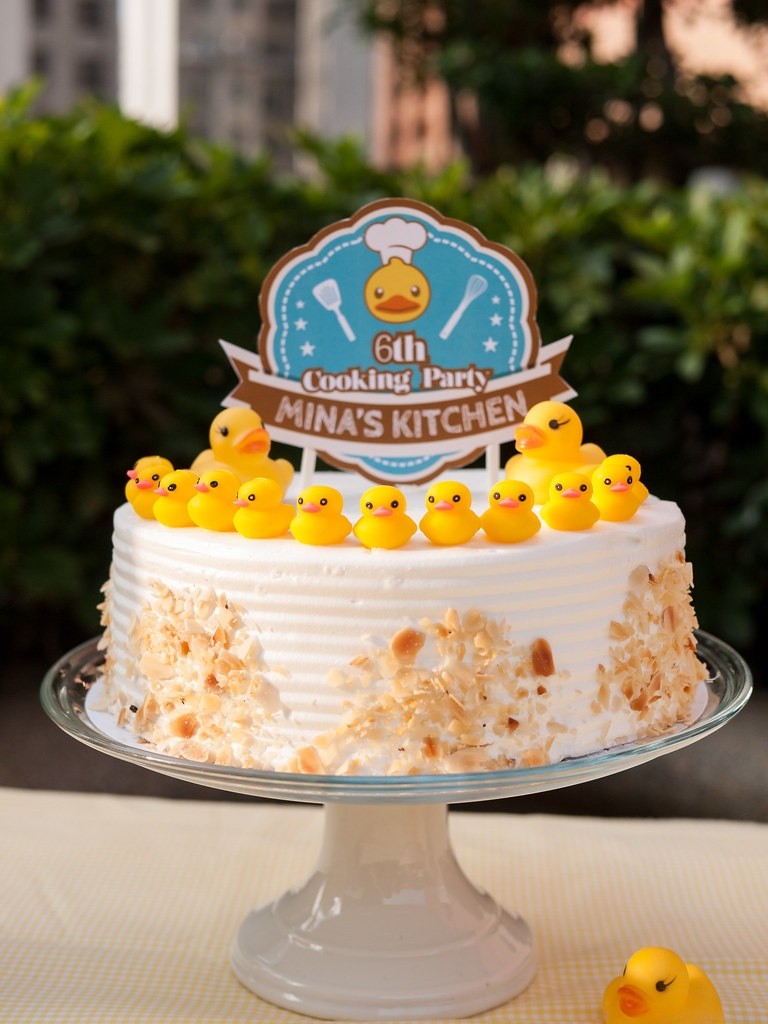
[96,469,709,776]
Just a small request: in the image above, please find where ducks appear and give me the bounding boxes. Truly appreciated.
[601,947,725,1024]
[122,401,649,547]
